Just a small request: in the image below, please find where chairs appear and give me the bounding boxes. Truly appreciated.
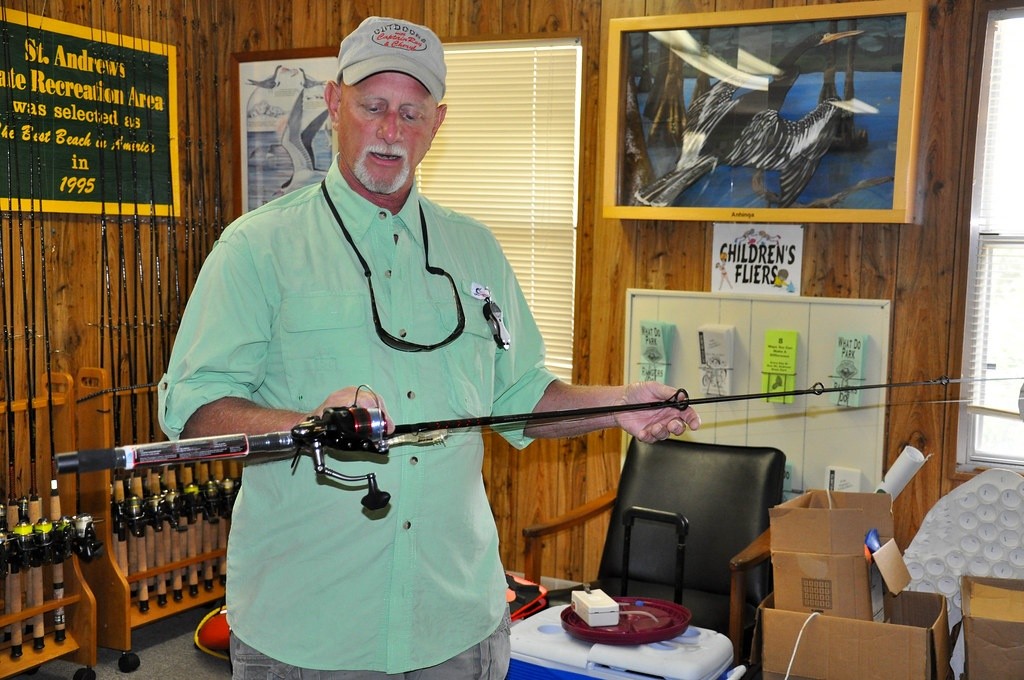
[522,436,786,680]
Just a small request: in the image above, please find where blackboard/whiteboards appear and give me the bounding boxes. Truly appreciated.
[620,284,890,510]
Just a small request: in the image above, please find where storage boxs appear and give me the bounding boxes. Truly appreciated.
[749,591,950,680]
[768,490,912,624]
[504,604,746,680]
[961,576,1024,680]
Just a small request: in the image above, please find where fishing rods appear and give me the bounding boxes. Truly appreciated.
[0,0,243,658]
[50,374,1024,510]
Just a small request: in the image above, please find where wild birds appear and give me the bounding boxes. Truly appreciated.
[247,65,326,89]
[271,90,331,195]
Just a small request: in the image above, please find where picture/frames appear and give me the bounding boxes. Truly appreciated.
[230,46,340,221]
[603,0,928,224]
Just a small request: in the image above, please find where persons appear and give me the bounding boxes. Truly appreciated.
[155,14,700,680]
